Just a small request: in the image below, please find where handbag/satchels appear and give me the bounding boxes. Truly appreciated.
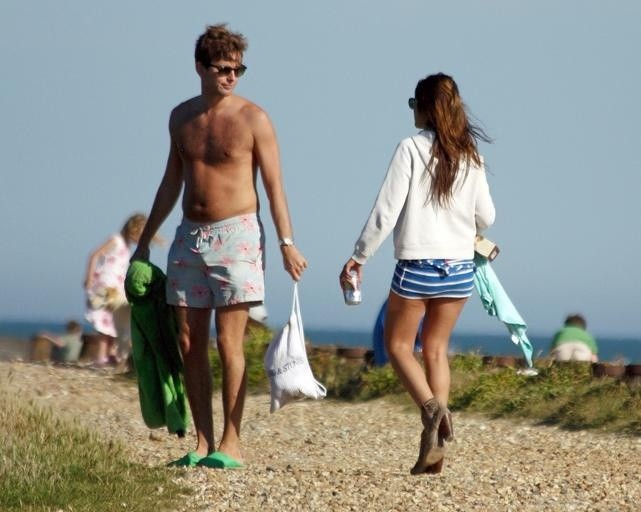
[264,313,325,414]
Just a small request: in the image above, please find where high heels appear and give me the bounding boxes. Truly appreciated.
[411,396,453,475]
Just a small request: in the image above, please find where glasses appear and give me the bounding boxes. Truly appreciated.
[209,62,246,77]
[409,98,418,108]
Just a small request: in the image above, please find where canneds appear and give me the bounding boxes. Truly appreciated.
[342,271,361,305]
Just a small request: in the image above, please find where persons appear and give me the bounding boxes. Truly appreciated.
[549,315,600,364]
[128,24,307,470]
[83,214,147,368]
[372,297,424,368]
[37,322,84,365]
[338,72,496,475]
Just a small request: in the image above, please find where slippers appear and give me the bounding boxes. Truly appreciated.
[198,452,246,468]
[167,450,204,466]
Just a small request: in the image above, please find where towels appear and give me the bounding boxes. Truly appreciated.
[473,251,534,369]
[125,258,189,438]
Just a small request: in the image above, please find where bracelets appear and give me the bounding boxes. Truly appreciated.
[277,238,294,246]
